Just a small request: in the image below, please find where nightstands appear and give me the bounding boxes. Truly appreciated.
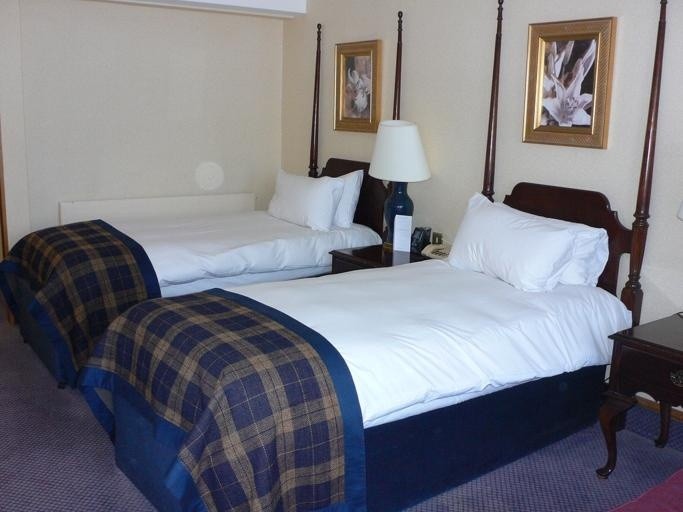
[329,241,445,274]
[596,309,683,480]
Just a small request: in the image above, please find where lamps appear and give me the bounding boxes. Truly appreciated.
[366,119,431,250]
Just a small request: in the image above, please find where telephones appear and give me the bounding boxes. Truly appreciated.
[421,244,452,260]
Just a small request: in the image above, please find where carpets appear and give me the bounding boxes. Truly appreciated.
[609,465,682,511]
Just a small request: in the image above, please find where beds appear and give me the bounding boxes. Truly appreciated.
[0,158,411,393]
[73,180,643,512]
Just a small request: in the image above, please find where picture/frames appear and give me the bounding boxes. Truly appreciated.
[521,15,616,151]
[332,38,383,133]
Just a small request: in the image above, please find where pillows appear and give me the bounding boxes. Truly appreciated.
[446,190,581,291]
[329,169,366,231]
[495,200,611,287]
[265,168,343,232]
